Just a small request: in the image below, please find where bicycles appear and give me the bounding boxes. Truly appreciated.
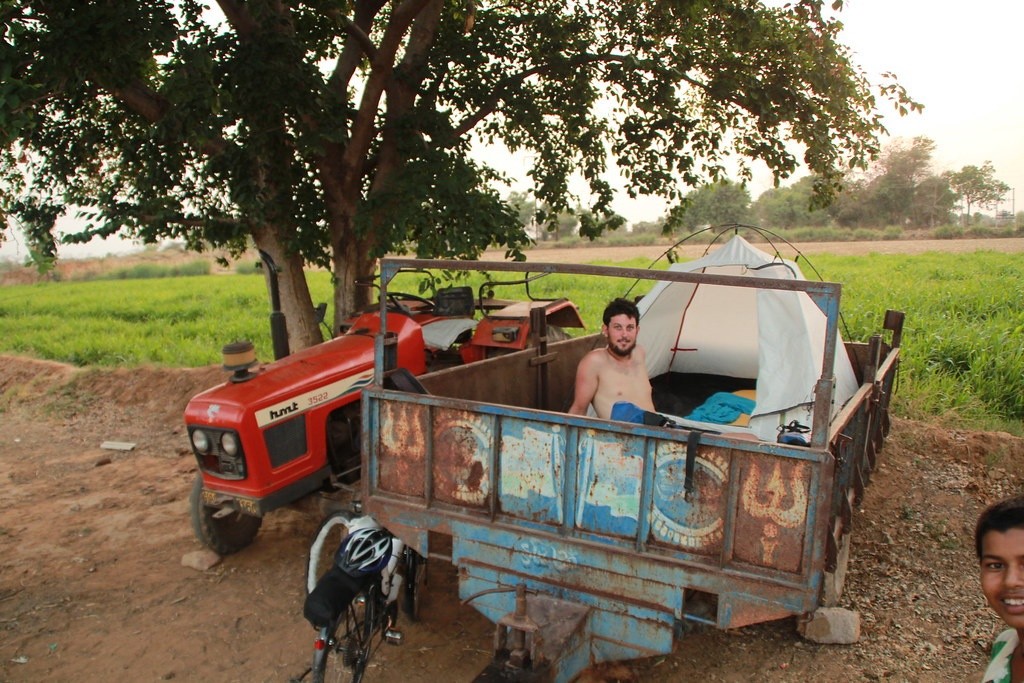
[290,501,428,683]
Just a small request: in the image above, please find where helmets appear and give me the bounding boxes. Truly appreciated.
[334,528,392,577]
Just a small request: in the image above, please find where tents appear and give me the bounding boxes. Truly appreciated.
[563,223,864,446]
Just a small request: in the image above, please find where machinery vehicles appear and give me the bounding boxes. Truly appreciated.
[178,267,588,558]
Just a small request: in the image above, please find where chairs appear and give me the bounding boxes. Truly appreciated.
[435,286,476,343]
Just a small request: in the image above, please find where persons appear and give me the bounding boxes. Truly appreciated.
[975,492,1024,683]
[569,298,758,442]
